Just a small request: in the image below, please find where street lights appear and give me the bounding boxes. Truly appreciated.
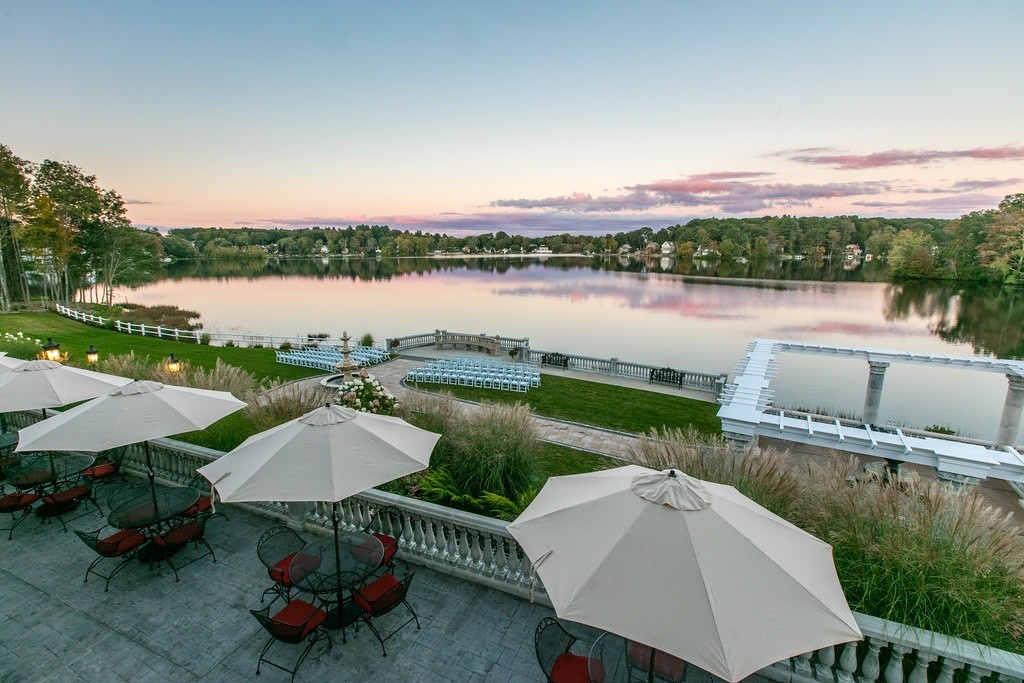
[43,337,60,361]
[86,345,99,371]
[168,353,180,373]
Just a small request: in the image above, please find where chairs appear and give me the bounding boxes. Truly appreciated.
[406,355,541,394]
[348,506,404,587]
[256,525,325,605]
[148,508,216,582]
[623,638,688,682]
[249,591,332,683]
[347,560,420,657]
[106,483,162,539]
[38,468,104,534]
[0,444,57,502]
[106,487,200,540]
[534,616,605,682]
[275,343,391,375]
[75,445,134,502]
[0,479,43,540]
[73,516,156,592]
[179,472,229,545]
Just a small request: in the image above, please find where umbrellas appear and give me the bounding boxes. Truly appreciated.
[13,377,248,533]
[0,360,135,494]
[506,462,865,683]
[195,402,444,608]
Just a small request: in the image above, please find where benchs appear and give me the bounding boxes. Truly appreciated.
[541,352,569,371]
[649,367,685,390]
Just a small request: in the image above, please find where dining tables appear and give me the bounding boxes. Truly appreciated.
[587,631,728,683]
[7,455,94,513]
[288,531,384,630]
